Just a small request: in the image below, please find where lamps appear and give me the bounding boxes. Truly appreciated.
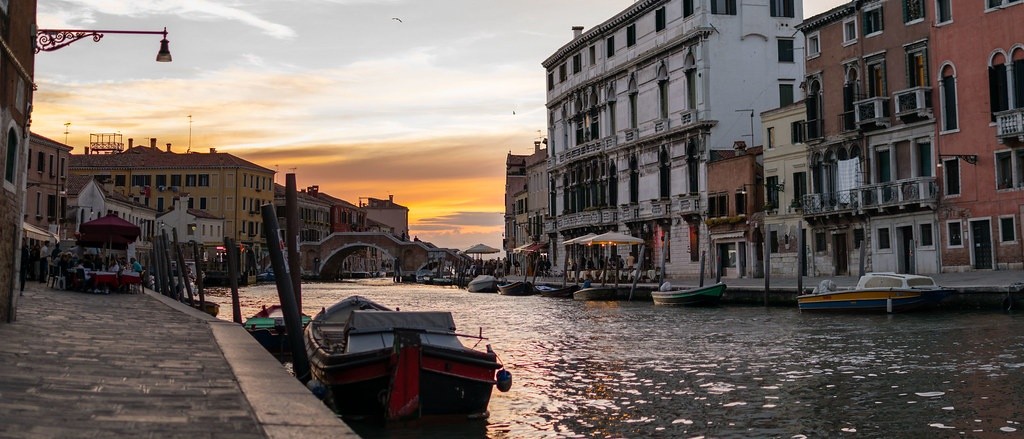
[31,24,172,62]
[937,155,977,168]
[66,204,94,215]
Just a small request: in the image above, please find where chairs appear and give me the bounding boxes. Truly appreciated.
[139,270,147,294]
[77,268,88,290]
[52,264,65,290]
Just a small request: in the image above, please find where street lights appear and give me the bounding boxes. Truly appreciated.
[734,109,755,147]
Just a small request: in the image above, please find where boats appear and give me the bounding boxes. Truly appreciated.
[468,274,498,293]
[245,294,512,422]
[497,281,533,295]
[572,287,618,301]
[651,282,726,307]
[535,285,576,297]
[796,272,960,314]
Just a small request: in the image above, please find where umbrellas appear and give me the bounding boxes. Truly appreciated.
[457,242,500,262]
[75,212,140,263]
[562,230,645,286]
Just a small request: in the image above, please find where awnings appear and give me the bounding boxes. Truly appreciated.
[22,222,60,244]
[514,243,545,253]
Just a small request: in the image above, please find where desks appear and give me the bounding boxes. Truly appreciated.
[87,271,141,292]
[566,270,658,283]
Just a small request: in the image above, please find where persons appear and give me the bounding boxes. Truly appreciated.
[343,224,419,242]
[20,237,142,297]
[459,252,636,282]
